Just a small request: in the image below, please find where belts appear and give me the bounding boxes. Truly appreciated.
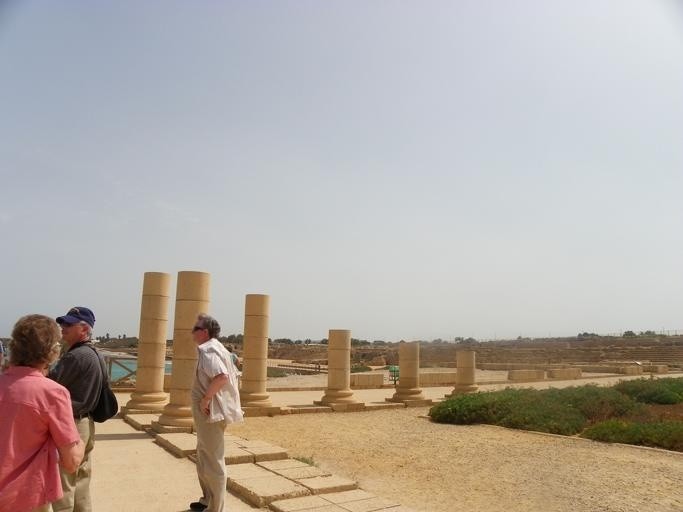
[74,412,94,419]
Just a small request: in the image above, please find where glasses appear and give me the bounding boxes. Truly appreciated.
[60,320,74,327]
[193,326,203,330]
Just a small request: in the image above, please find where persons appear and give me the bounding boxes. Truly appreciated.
[0,314,86,512]
[43,306,107,512]
[189,314,244,512]
[0,341,4,368]
[224,343,241,369]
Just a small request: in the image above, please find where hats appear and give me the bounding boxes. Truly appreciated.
[56,307,95,329]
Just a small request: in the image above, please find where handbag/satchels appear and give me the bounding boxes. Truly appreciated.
[92,380,118,422]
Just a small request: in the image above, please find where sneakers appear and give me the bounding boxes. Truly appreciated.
[190,502,207,512]
